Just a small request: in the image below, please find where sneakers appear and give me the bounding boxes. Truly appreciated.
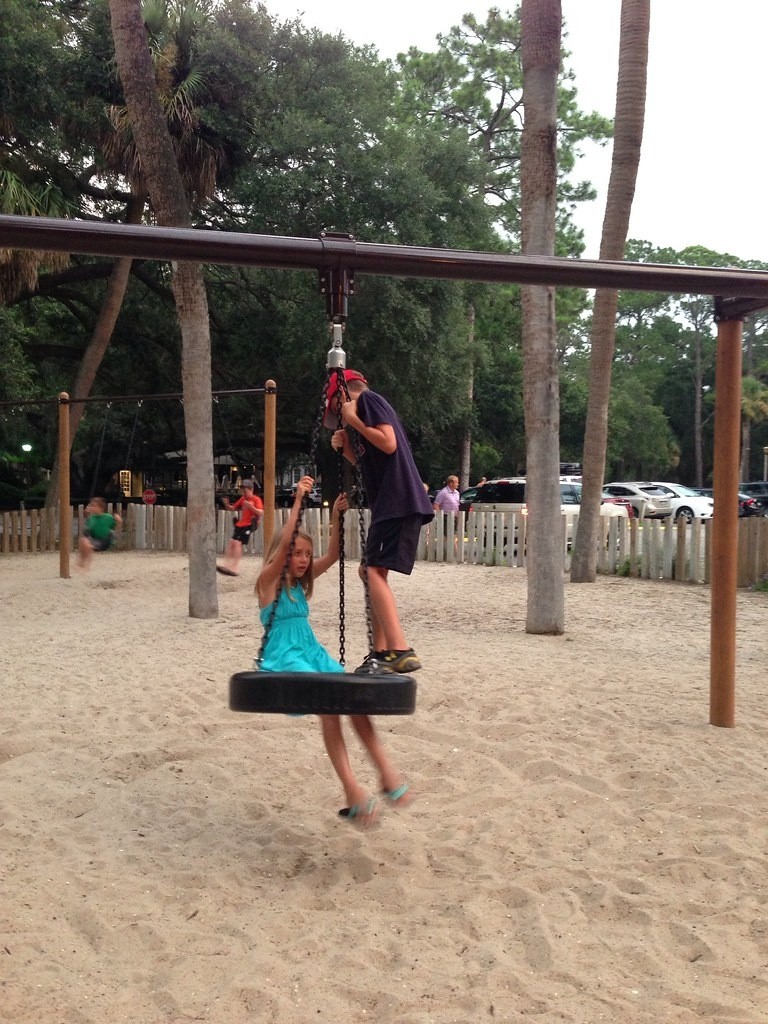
[354,648,394,674]
[368,647,421,673]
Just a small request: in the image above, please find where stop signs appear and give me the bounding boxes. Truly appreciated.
[142,490,156,505]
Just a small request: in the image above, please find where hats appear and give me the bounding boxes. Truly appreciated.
[315,357,367,430]
[238,479,254,488]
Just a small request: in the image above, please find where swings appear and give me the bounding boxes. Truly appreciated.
[213,396,257,531]
[228,330,419,716]
[83,402,143,552]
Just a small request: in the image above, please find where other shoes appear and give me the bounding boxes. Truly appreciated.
[215,564,240,577]
[339,797,380,819]
[381,785,414,808]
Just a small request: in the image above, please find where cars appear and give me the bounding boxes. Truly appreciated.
[696,488,764,517]
[602,491,634,520]
[458,487,481,520]
[275,485,323,509]
[649,482,714,524]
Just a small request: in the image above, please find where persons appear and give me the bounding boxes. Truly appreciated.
[216,479,263,575]
[477,477,487,486]
[253,477,415,829]
[75,497,122,571]
[433,475,460,512]
[322,369,435,673]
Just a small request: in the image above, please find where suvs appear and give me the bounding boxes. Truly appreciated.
[468,475,630,553]
[602,482,673,522]
